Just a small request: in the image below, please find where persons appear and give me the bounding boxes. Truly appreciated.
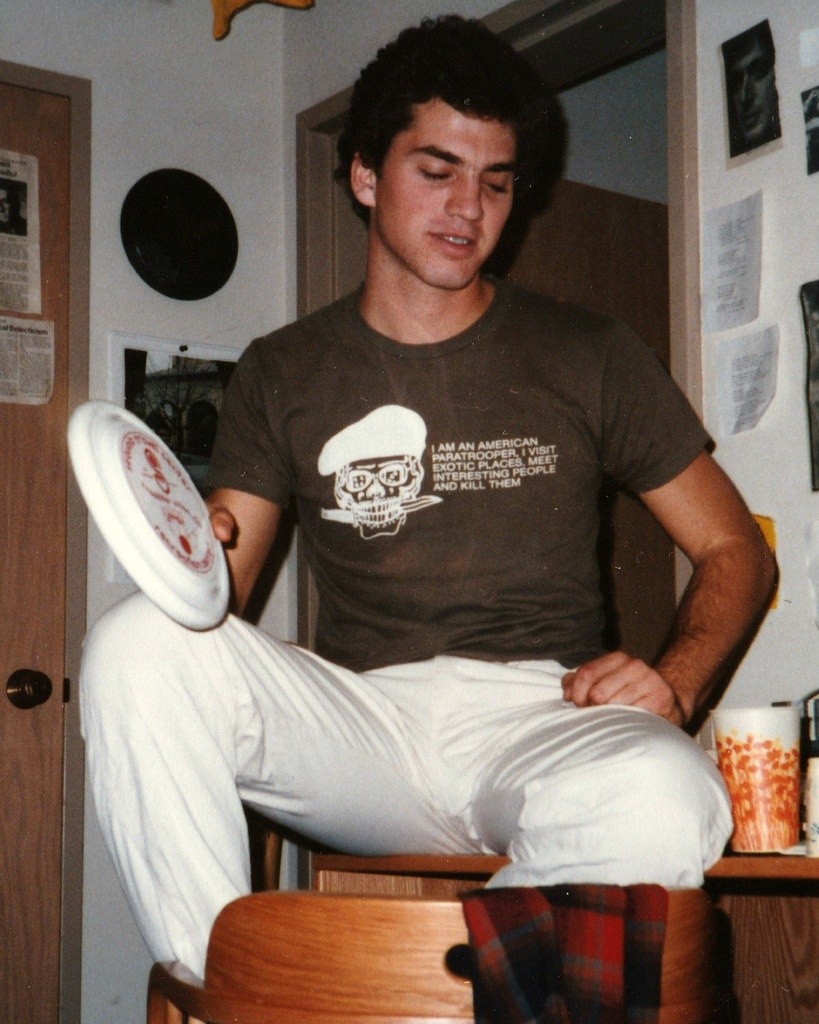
[724,19,781,156]
[78,15,781,990]
[0,178,27,237]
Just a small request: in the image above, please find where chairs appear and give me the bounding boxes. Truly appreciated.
[147,893,740,1024]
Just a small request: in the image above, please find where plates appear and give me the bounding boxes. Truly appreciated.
[66,399,230,631]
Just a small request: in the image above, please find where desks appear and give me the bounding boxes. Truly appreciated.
[294,854,819,1024]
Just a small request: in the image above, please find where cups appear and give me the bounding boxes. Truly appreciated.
[709,710,800,853]
